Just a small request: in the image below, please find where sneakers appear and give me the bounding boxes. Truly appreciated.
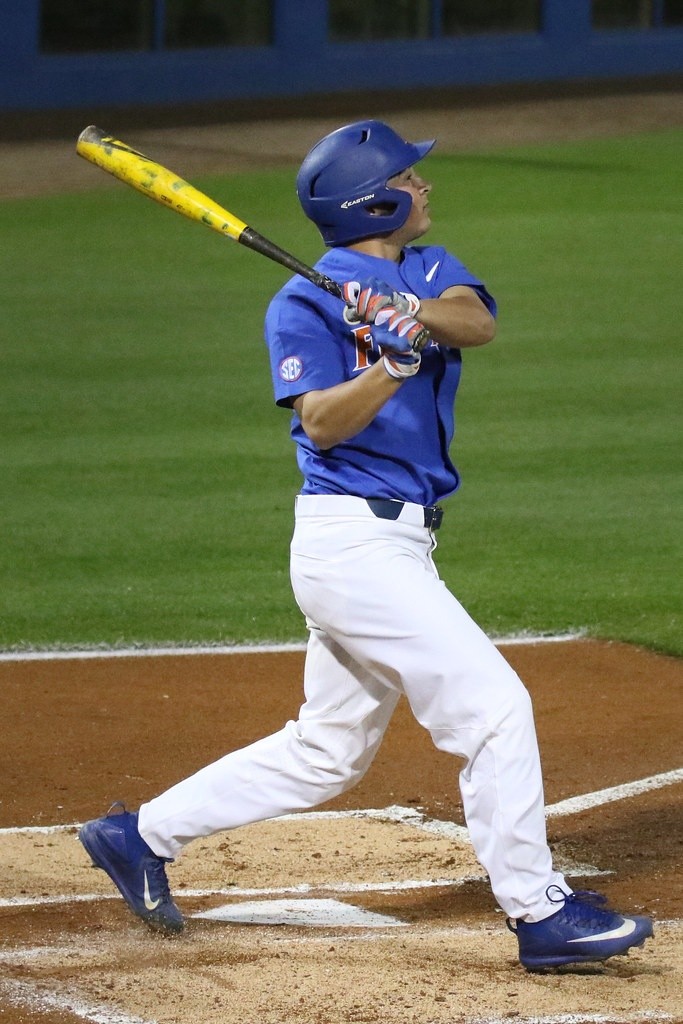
[77,800,183,938]
[505,896,654,971]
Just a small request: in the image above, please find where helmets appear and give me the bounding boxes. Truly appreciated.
[295,119,437,246]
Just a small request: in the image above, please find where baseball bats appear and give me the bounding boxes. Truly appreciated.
[74,125,431,353]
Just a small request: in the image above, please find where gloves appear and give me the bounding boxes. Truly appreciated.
[338,270,422,379]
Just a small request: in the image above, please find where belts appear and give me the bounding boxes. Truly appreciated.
[293,493,443,530]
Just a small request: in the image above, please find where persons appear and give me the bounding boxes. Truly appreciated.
[77,124,653,970]
